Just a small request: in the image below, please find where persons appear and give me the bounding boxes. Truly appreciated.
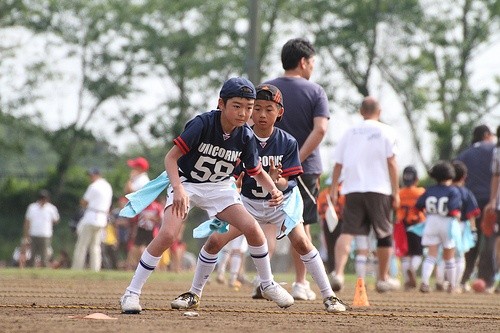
[395,125,500,295]
[120,77,294,314]
[215,234,248,289]
[72,168,114,271]
[172,84,352,312]
[13,191,71,268]
[103,158,185,271]
[318,96,403,292]
[252,38,329,302]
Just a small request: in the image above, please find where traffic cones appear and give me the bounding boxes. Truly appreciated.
[352,278,371,306]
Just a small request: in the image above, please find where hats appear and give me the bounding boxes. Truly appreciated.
[451,160,466,177]
[427,160,457,179]
[402,166,416,185]
[252,82,286,124]
[128,156,150,175]
[215,77,258,111]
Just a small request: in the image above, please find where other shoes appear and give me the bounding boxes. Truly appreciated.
[407,277,497,293]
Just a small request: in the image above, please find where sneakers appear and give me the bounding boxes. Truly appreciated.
[327,271,344,291]
[252,276,295,308]
[171,292,202,310]
[119,290,144,313]
[322,297,346,313]
[293,280,316,301]
[377,277,400,290]
[212,272,254,290]
